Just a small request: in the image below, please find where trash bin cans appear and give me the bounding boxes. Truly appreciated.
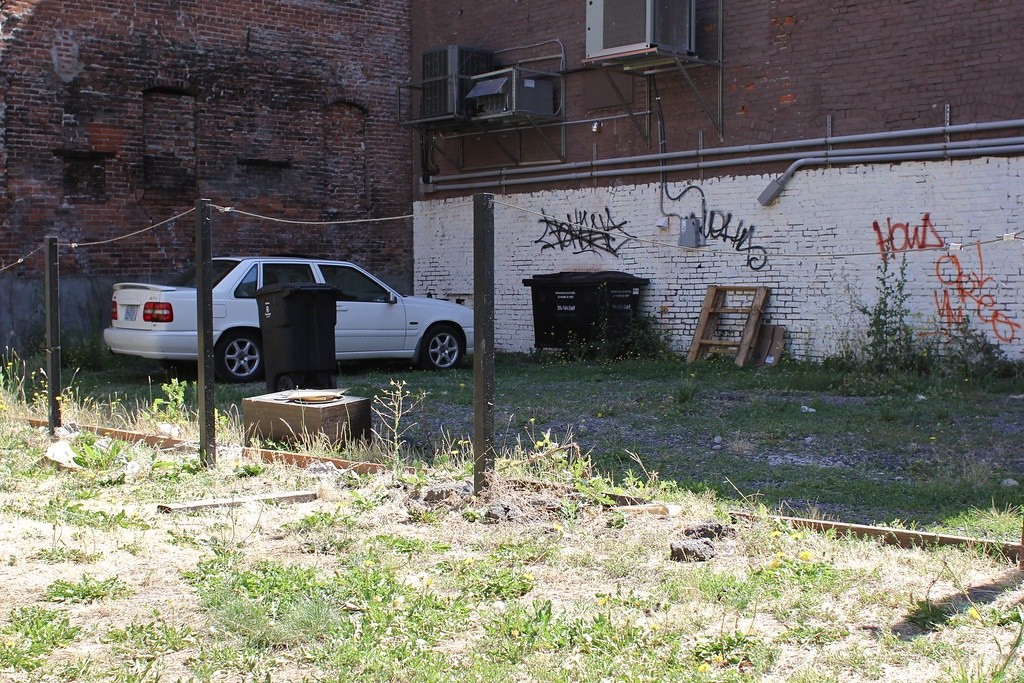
[252,281,338,395]
[522,271,651,349]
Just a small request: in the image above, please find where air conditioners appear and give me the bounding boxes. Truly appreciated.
[465,76,553,114]
[420,45,495,118]
[584,0,696,60]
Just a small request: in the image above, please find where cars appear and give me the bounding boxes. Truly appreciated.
[104,255,476,385]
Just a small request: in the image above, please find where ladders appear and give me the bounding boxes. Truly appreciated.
[686,284,770,369]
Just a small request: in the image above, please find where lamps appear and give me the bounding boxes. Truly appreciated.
[655,217,669,230]
[591,122,602,133]
